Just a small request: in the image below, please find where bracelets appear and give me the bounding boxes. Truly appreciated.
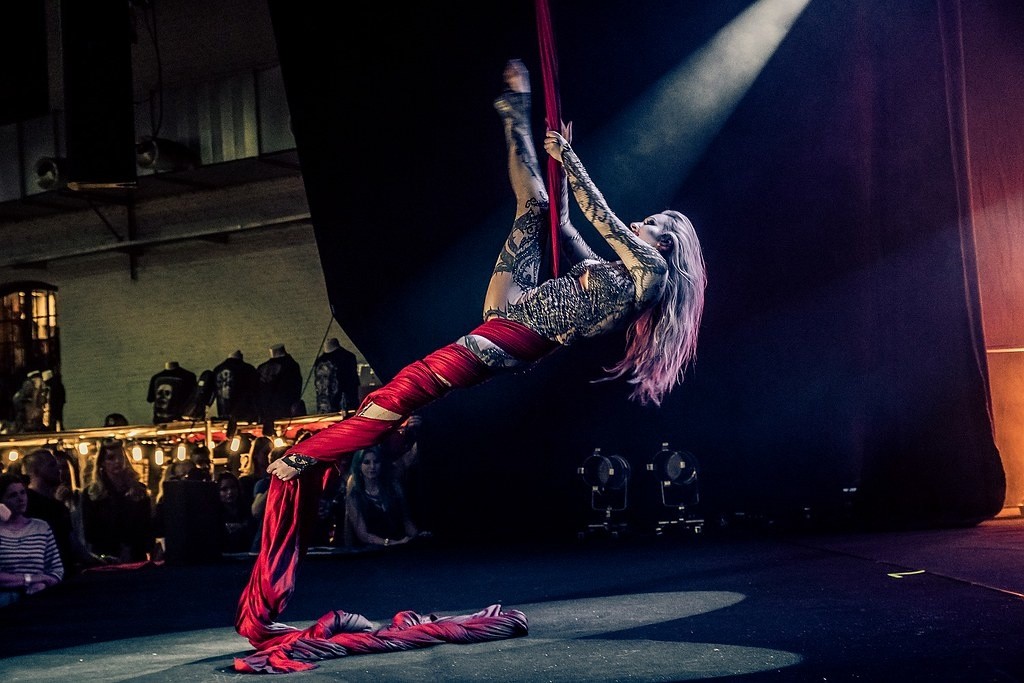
[95,554,105,560]
[24,573,33,587]
[383,539,389,547]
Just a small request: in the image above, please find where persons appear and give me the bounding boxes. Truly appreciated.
[146,361,199,426]
[263,57,709,483]
[0,417,431,633]
[255,343,304,421]
[213,351,258,421]
[35,370,66,433]
[313,337,359,414]
[18,371,44,432]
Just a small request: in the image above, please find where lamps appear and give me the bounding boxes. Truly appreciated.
[644,437,707,540]
[567,445,635,543]
